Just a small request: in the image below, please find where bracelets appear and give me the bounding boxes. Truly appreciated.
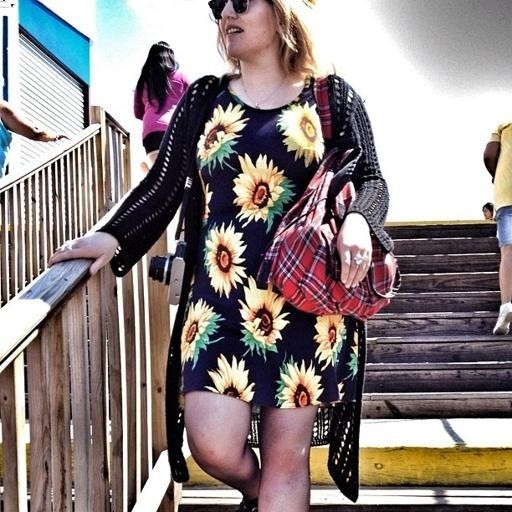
[54,135,59,141]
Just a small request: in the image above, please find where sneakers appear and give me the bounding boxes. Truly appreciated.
[492,302,512,335]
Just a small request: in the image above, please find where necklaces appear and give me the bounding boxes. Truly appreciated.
[240,70,288,110]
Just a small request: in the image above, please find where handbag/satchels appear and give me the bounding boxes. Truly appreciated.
[254,76,402,324]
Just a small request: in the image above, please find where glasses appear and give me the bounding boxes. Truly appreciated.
[208,0,249,20]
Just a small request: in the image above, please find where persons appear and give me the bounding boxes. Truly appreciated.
[132,40,190,165]
[46,1,391,512]
[487,121,512,336]
[482,202,494,220]
[0,97,70,180]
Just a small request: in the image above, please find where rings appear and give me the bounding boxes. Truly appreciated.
[353,254,363,264]
[65,243,72,250]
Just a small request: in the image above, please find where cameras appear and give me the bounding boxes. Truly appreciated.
[148,241,187,306]
